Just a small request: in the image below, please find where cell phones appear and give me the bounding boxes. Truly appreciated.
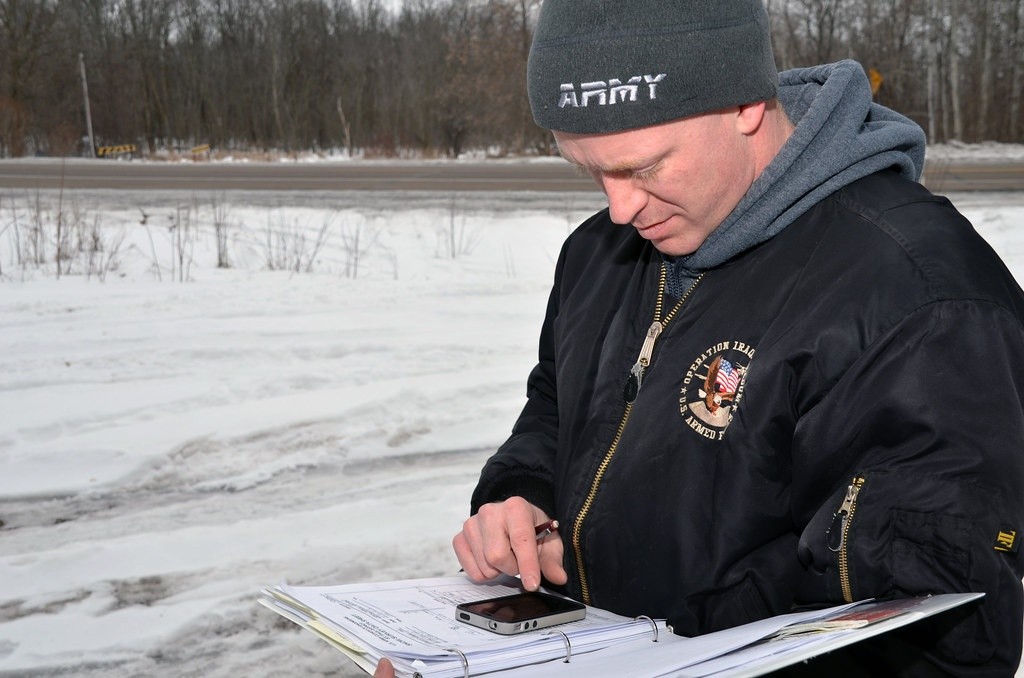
[454,590,587,636]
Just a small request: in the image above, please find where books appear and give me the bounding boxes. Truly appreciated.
[257,570,988,677]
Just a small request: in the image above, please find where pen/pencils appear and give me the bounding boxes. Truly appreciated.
[456,519,560,574]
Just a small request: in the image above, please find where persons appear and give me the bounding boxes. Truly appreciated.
[376,0,1024,678]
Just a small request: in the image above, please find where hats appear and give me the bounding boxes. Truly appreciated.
[527,0,779,133]
